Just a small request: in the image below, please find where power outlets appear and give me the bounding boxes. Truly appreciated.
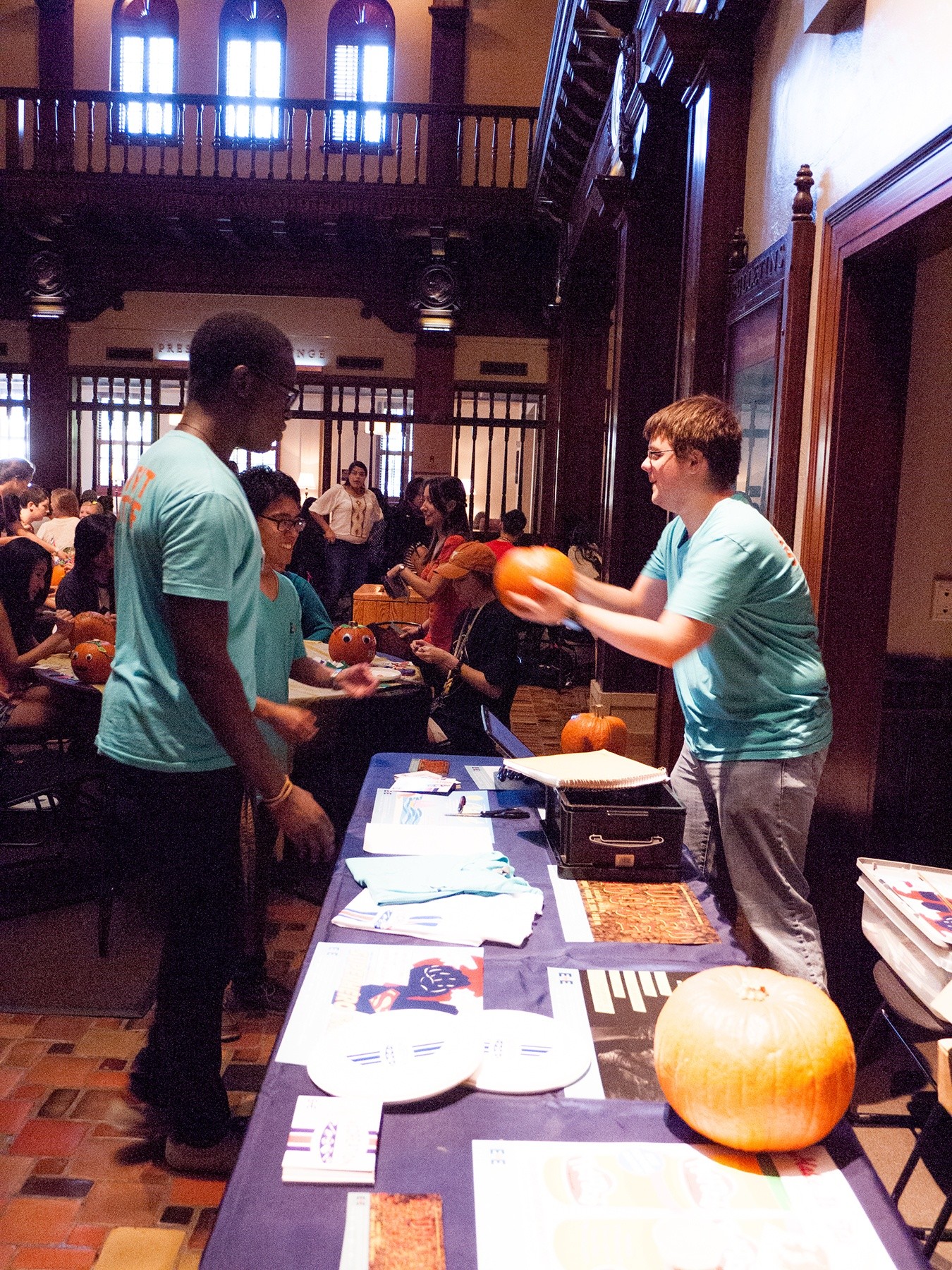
[931,578,951,624]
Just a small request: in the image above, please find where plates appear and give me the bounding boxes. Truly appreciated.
[369,667,401,681]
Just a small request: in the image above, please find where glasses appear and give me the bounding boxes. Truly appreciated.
[20,478,32,488]
[258,513,306,532]
[255,369,301,408]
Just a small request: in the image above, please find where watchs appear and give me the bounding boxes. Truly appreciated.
[452,661,464,675]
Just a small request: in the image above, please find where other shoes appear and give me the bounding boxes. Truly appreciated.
[163,1129,243,1171]
[233,979,291,1014]
[121,1073,154,1116]
[220,1002,240,1040]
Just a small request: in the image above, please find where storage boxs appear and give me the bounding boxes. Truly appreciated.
[544,782,687,885]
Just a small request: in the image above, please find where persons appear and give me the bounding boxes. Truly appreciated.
[239,464,379,1010]
[308,460,385,592]
[0,455,118,617]
[299,478,604,590]
[0,537,65,729]
[411,543,521,753]
[95,313,337,1173]
[507,397,836,999]
[387,479,472,668]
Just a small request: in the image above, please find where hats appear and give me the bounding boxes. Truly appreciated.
[80,490,98,500]
[432,540,495,579]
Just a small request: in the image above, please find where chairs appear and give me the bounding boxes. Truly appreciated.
[0,667,144,919]
[851,956,952,1208]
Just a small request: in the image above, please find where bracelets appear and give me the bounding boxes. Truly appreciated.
[420,623,424,636]
[261,774,291,804]
[266,783,294,808]
[331,669,340,689]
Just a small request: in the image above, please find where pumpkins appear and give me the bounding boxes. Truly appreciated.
[561,704,629,757]
[493,545,576,613]
[653,965,856,1151]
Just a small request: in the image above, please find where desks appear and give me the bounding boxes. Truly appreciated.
[196,750,931,1270]
[30,637,424,962]
[351,583,429,635]
